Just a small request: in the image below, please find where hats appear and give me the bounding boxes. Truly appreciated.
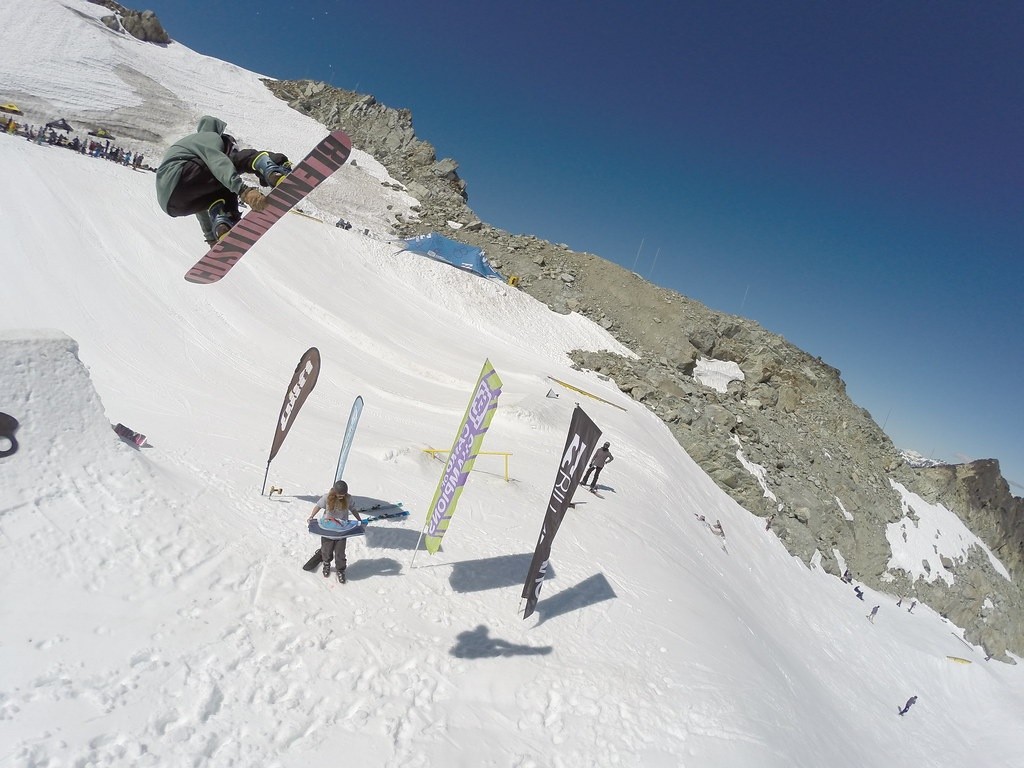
[604,442,610,447]
[334,481,348,494]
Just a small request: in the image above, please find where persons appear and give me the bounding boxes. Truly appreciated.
[154,116,294,249]
[856,592,864,600]
[0,113,145,171]
[306,481,364,584]
[335,217,353,231]
[844,570,853,583]
[908,600,917,612]
[766,514,776,531]
[896,598,902,607]
[580,441,614,494]
[899,695,918,716]
[853,585,862,593]
[869,605,880,621]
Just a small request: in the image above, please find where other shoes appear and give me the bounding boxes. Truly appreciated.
[323,561,330,577]
[580,481,586,485]
[337,570,346,583]
[590,486,594,491]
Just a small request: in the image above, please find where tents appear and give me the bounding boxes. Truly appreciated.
[0,102,25,116]
[385,231,509,288]
[87,126,116,140]
[45,117,75,137]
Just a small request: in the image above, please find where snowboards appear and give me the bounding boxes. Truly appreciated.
[184,130,352,285]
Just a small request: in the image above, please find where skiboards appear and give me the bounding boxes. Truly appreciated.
[695,514,725,538]
[348,502,411,522]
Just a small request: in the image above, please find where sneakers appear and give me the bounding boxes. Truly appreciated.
[252,151,291,188]
[207,198,233,240]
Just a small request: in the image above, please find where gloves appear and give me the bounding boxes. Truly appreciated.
[207,241,217,249]
[590,462,592,467]
[241,187,269,212]
